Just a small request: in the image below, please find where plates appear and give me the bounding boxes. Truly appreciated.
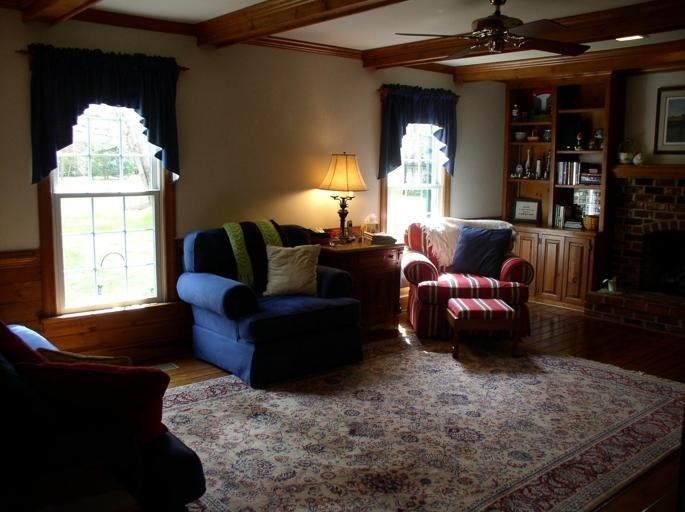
[527,136,541,142]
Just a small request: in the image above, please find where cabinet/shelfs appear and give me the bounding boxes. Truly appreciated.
[501,75,612,232]
[319,242,406,334]
[508,232,538,298]
[535,234,592,306]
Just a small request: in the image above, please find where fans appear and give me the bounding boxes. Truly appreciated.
[393,0,594,58]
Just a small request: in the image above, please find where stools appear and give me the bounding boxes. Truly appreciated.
[446,296,517,359]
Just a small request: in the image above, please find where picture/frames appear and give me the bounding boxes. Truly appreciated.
[652,84,685,156]
[512,197,542,226]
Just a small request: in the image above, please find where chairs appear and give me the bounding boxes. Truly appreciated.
[176,222,363,392]
[400,219,536,338]
[1,318,206,511]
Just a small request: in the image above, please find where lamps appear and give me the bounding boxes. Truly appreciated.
[317,152,368,242]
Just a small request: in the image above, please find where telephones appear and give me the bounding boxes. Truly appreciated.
[347,220,356,241]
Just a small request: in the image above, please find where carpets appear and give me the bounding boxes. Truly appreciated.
[161,341,685,512]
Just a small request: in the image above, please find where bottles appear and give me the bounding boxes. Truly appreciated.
[511,104,519,121]
[543,130,552,142]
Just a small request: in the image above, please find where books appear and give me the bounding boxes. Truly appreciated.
[555,161,601,228]
[364,230,396,244]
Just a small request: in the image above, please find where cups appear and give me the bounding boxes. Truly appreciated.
[538,160,546,178]
[366,223,380,246]
[607,280,623,295]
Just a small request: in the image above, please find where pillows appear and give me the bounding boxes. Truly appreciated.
[36,346,134,370]
[15,358,171,453]
[262,242,321,298]
[449,221,515,281]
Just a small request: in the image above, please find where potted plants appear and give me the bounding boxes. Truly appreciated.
[615,136,637,163]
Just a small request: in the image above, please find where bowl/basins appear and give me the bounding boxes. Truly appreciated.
[514,131,528,141]
